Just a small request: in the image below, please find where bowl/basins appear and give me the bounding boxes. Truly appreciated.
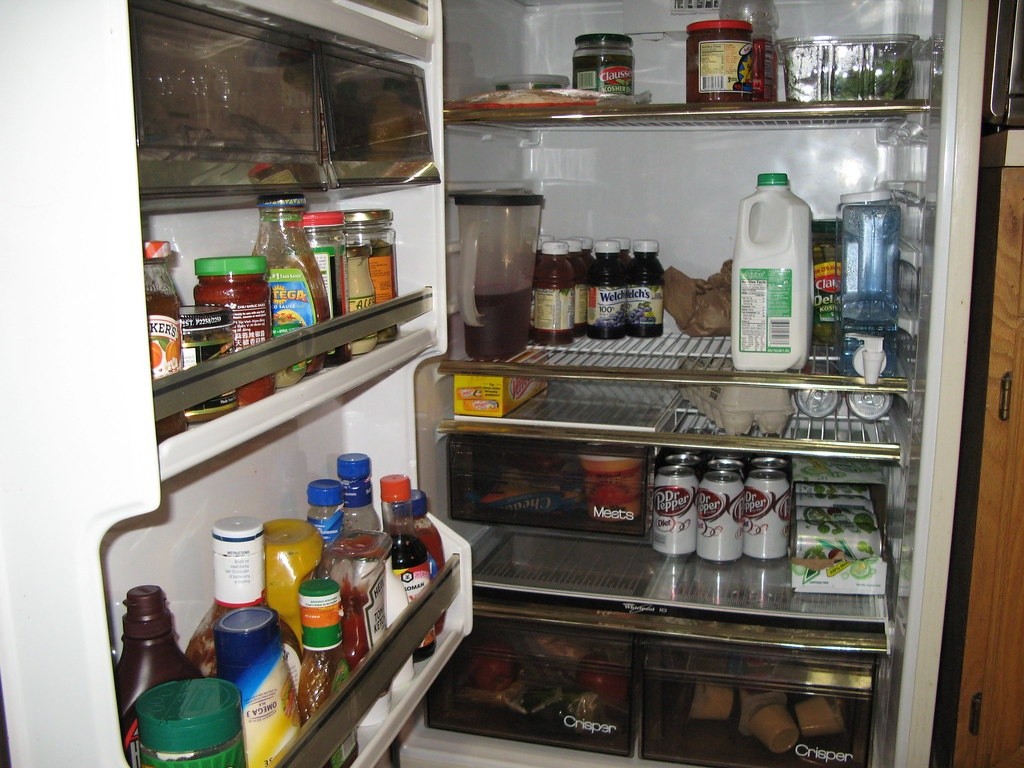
[492,74,569,89]
[774,34,919,102]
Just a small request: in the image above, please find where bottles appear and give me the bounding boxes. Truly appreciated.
[144,241,189,445]
[193,257,275,406]
[627,240,664,337]
[345,245,376,355]
[185,517,393,768]
[285,211,352,367]
[813,218,845,346]
[572,33,633,96]
[253,195,329,387]
[528,236,595,344]
[338,454,382,533]
[115,586,246,768]
[719,0,778,101]
[380,474,445,691]
[586,238,632,340]
[731,173,814,370]
[344,209,397,343]
[686,20,752,101]
[180,306,238,423]
[306,479,345,547]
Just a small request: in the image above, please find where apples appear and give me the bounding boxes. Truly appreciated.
[580,653,628,704]
[472,638,515,693]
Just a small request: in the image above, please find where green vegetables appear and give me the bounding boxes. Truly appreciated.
[784,53,911,102]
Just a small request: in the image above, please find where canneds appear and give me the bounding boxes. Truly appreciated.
[685,19,754,103]
[194,255,275,406]
[142,241,186,442]
[652,449,792,565]
[346,245,378,355]
[179,305,239,423]
[573,33,634,96]
[248,162,301,184]
[794,362,841,419]
[303,211,353,367]
[813,219,845,347]
[846,391,894,419]
[346,209,399,342]
[134,678,247,768]
[794,481,882,560]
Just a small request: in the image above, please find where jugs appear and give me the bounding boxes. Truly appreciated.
[446,186,543,358]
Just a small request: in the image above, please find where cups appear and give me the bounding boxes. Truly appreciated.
[580,454,640,519]
[686,681,842,753]
